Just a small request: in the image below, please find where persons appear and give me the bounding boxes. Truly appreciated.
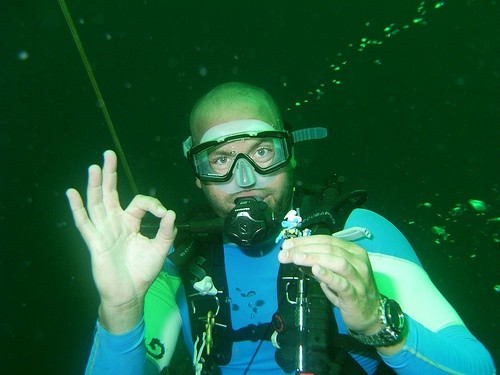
[64,82,497,375]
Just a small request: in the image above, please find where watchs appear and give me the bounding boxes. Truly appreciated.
[347,295,406,347]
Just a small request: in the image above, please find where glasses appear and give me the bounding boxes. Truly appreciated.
[187,130,294,183]
